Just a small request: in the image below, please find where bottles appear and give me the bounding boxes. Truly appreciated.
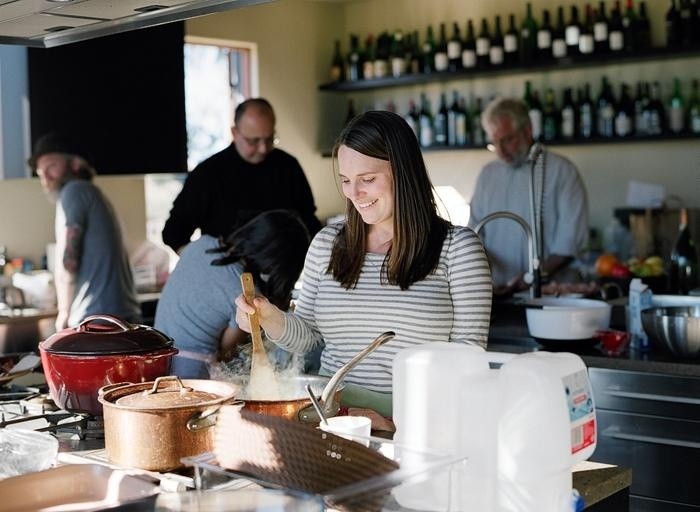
[335,74,700,149]
[331,1,700,83]
[671,208,694,296]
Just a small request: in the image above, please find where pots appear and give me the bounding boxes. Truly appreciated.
[212,330,396,420]
[37,312,178,415]
[97,375,239,471]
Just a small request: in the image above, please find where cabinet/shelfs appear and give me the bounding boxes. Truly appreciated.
[318,39,700,158]
[584,356,700,512]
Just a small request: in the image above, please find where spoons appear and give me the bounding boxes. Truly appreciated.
[237,273,279,397]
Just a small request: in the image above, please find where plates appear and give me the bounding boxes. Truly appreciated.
[609,296,700,324]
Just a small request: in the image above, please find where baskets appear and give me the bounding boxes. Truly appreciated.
[215,406,398,512]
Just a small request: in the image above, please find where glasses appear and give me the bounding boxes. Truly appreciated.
[236,129,277,145]
[487,120,528,152]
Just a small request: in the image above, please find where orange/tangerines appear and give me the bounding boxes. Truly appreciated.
[634,264,654,276]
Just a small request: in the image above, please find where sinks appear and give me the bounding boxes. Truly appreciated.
[484,322,544,354]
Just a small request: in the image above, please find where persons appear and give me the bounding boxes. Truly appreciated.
[464,98,591,299]
[27,134,143,331]
[234,111,494,433]
[161,98,323,256]
[154,209,311,380]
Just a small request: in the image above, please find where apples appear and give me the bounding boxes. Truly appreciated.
[613,263,630,277]
[627,257,642,267]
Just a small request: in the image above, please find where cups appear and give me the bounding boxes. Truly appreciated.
[320,415,373,449]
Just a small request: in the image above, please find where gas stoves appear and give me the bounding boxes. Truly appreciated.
[0,382,257,491]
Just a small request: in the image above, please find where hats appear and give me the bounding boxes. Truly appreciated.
[29,135,95,172]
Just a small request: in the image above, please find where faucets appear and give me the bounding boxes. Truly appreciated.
[475,213,536,296]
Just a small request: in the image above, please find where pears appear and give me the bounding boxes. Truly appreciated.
[651,267,663,276]
[645,257,663,268]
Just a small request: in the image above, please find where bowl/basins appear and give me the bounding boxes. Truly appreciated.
[0,429,59,476]
[0,464,161,512]
[594,330,631,354]
[526,298,613,341]
[641,306,700,354]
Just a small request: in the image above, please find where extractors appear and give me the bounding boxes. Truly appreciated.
[0,0,280,48]
